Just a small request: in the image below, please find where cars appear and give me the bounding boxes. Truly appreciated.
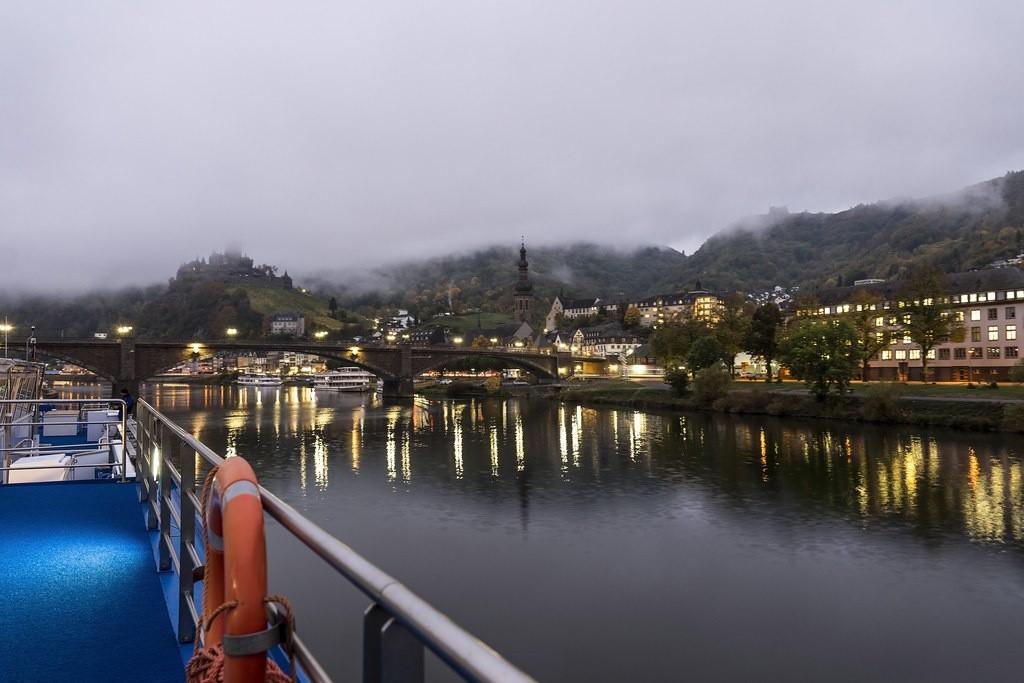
[440,379,452,385]
[513,380,531,385]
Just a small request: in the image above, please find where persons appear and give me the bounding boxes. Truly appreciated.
[117,388,134,421]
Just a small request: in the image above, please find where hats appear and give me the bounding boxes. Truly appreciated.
[120,388,129,394]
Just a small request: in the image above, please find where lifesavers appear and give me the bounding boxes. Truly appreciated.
[198,455,270,683]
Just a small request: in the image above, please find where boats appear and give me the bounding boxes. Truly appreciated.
[313,366,378,392]
[237,373,281,386]
